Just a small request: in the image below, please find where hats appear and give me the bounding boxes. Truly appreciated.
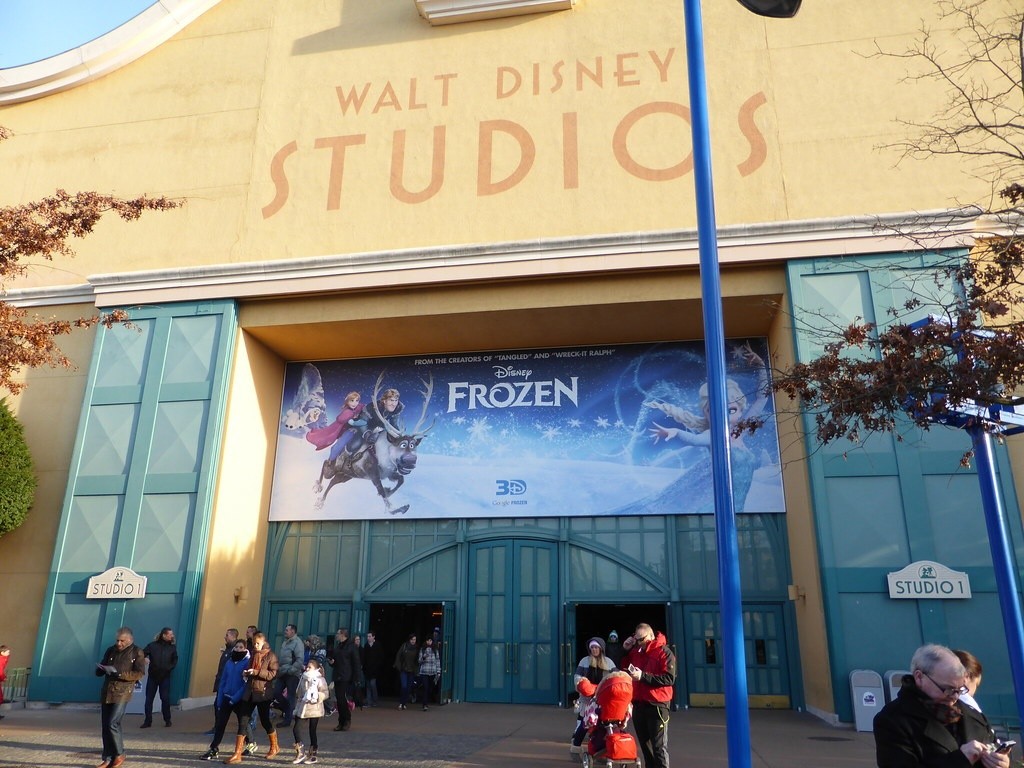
[589,640,602,651]
[610,630,618,639]
[434,627,441,632]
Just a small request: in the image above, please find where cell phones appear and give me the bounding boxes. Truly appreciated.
[995,741,1016,754]
[628,663,637,674]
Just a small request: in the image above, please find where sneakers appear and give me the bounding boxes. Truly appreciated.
[200,749,219,759]
[241,742,258,756]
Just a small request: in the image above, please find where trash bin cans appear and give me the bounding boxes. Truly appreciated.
[125,661,162,714]
[849,669,885,732]
[883,670,913,704]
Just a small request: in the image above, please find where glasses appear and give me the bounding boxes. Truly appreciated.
[915,669,969,697]
[635,635,648,642]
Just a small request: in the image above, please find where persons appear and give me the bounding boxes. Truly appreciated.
[394,627,441,711]
[95,627,145,768]
[199,624,380,765]
[873,644,1015,768]
[570,623,676,768]
[0,645,12,720]
[140,627,178,729]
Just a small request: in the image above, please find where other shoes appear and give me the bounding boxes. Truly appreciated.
[423,704,429,710]
[277,721,290,727]
[325,707,336,717]
[204,728,215,735]
[166,721,171,727]
[141,722,151,728]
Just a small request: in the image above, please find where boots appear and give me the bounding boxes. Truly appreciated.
[304,746,318,764]
[265,729,281,759]
[342,717,351,731]
[292,741,307,764]
[223,735,246,764]
[335,722,343,730]
[570,742,583,763]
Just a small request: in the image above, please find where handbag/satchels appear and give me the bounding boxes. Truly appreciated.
[598,733,637,760]
[392,643,407,673]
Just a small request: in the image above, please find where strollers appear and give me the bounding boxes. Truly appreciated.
[582,671,641,768]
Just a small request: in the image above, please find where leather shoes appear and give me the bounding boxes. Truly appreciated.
[110,752,126,768]
[96,760,113,768]
